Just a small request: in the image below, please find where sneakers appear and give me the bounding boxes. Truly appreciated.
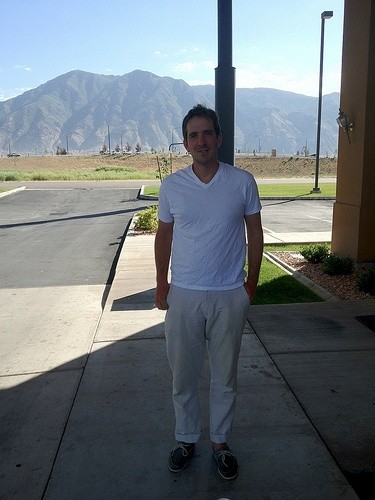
[167,442,195,473]
[211,445,239,480]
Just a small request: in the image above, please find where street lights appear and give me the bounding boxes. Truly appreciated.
[311,7,333,194]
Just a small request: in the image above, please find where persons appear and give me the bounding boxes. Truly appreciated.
[152,104,263,480]
